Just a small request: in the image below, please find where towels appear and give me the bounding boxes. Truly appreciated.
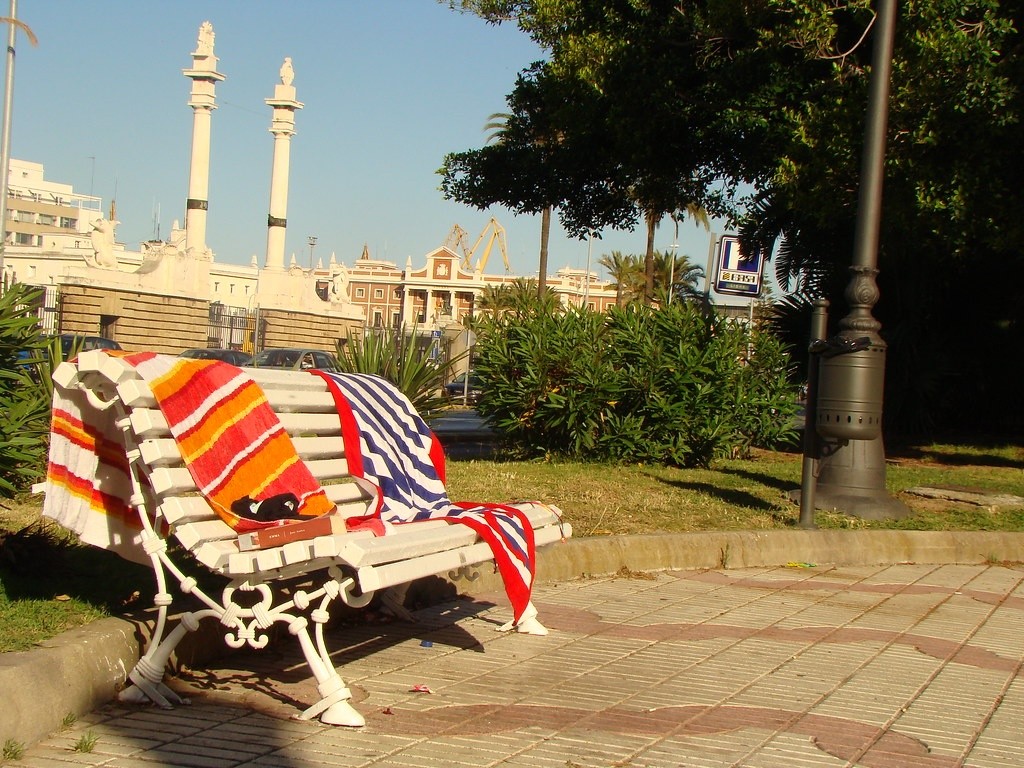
[40,345,340,571]
[301,361,550,637]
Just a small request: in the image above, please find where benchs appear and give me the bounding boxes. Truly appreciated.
[32,348,575,728]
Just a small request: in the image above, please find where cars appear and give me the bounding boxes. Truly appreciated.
[245,348,341,373]
[16,334,122,382]
[175,349,254,367]
[441,370,485,406]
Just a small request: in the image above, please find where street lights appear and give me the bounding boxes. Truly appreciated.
[668,244,679,305]
